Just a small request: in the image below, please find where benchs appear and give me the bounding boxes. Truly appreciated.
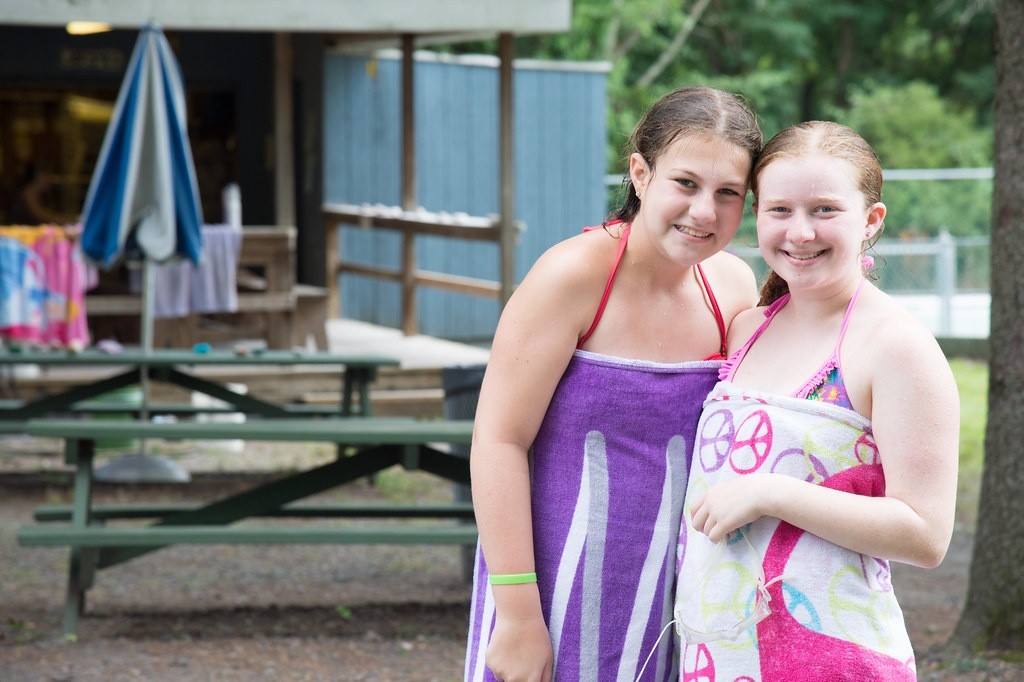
[2,225,480,640]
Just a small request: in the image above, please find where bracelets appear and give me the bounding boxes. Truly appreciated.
[489,572,538,587]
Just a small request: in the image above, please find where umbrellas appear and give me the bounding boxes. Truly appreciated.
[81,21,204,484]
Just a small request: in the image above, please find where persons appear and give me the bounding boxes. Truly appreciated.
[463,86,760,681]
[665,117,961,682]
